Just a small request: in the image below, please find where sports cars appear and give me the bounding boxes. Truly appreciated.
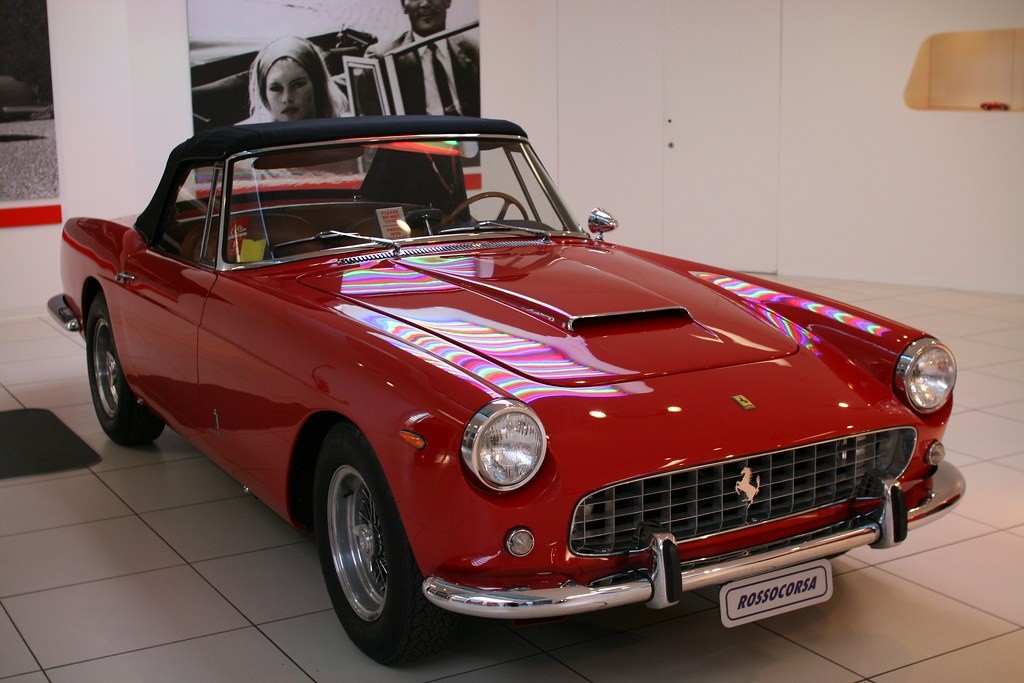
[45,117,969,664]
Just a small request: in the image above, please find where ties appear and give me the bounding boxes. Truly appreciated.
[427,44,461,115]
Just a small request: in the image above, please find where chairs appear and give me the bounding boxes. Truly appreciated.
[182,215,324,268]
[337,207,455,249]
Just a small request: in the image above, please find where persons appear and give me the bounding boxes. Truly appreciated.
[230,33,361,174]
[327,0,479,118]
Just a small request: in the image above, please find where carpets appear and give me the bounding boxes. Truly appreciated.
[0,408,101,480]
